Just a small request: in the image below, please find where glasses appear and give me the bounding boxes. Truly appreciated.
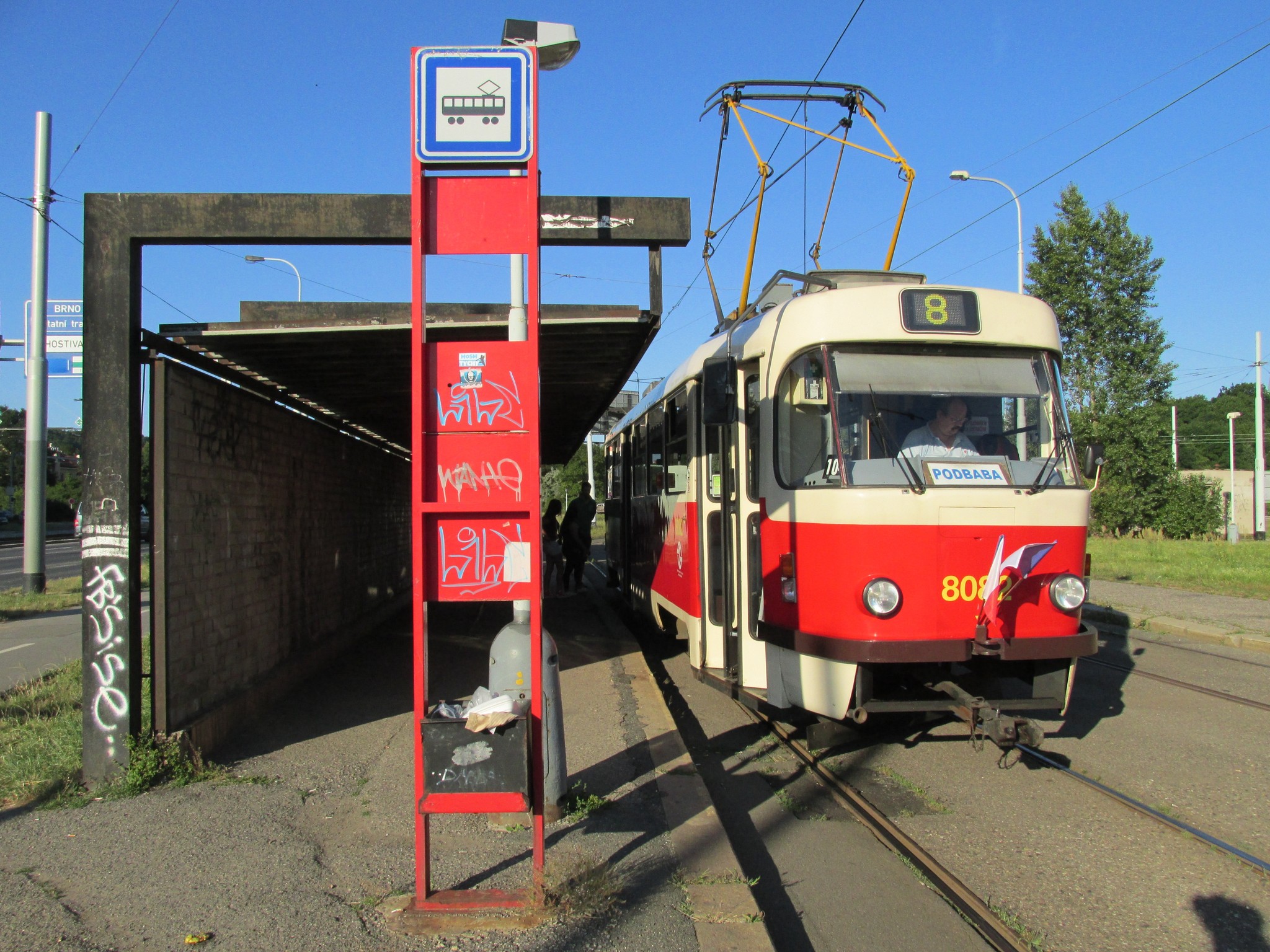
[948,413,968,423]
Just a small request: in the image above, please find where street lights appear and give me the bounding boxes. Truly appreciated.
[246,255,303,301]
[950,169,1025,295]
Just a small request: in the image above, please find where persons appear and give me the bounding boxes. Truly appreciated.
[541,499,577,600]
[560,482,597,591]
[897,397,982,458]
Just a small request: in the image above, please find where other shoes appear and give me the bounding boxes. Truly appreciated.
[563,589,570,595]
[556,590,570,598]
[577,587,587,593]
[543,590,555,598]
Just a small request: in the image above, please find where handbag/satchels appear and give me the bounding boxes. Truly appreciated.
[543,536,563,557]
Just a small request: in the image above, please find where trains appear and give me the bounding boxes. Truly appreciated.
[593,78,1105,755]
